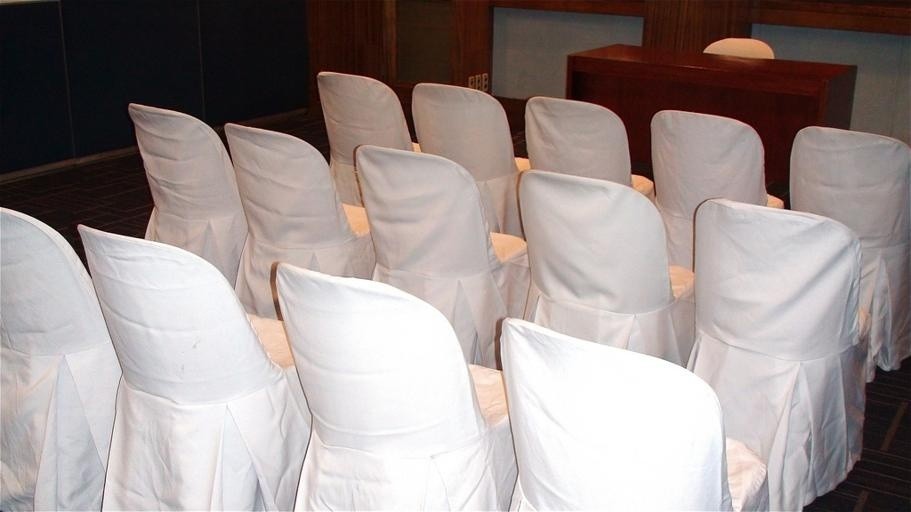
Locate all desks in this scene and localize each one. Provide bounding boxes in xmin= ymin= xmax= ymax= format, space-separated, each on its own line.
xmin=565 ymin=44 xmax=857 ymax=209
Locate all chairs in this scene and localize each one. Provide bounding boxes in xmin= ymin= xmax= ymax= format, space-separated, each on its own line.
xmin=703 ymin=38 xmax=775 ymax=60
